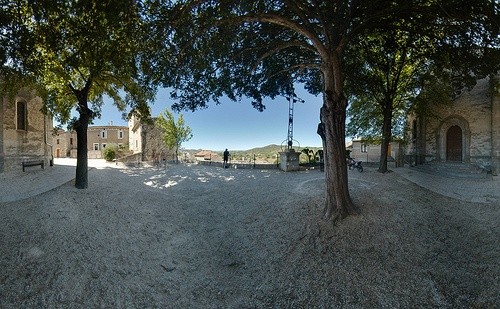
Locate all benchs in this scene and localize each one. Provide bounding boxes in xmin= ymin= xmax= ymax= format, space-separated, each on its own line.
xmin=22 ymin=160 xmax=44 ymax=172
xmin=300 ymin=161 xmax=320 ymax=169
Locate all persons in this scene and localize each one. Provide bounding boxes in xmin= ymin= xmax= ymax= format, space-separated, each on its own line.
xmin=223 ymin=148 xmax=229 ymax=168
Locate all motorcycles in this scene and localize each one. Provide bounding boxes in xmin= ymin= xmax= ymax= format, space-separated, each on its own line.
xmin=348 ymin=157 xmax=364 ymax=173
